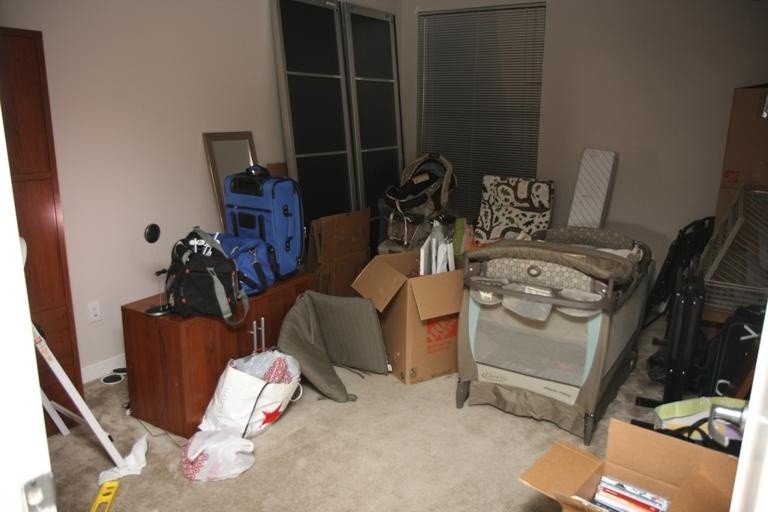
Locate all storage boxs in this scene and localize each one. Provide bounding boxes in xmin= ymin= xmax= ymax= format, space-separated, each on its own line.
xmin=517 ymin=416 xmax=739 ymax=511
xmin=350 ymin=248 xmax=464 ymax=387
xmin=699 ymin=83 xmax=768 ymax=323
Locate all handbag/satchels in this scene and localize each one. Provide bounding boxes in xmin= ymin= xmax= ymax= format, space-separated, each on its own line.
xmin=383 ymin=152 xmax=458 ymax=224
xmin=196 ymin=349 xmax=303 ymax=438
xmin=211 ymin=231 xmax=277 ymax=295
xmin=164 ymin=236 xmax=238 ymax=319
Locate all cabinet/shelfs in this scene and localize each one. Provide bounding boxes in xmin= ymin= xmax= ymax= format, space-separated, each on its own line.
xmin=120 ymin=274 xmax=317 ymax=440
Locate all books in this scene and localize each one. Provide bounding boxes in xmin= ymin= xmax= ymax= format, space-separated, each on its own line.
xmin=593 ymin=474 xmax=671 ymax=512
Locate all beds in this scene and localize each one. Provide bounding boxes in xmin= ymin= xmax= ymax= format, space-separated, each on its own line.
xmin=456 ymin=226 xmax=652 ymax=448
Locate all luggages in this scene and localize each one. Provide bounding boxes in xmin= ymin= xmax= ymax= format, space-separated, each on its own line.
xmin=666 ymin=272 xmax=704 ymax=377
xmin=704 ymin=304 xmax=765 ymax=396
xmin=224 ymin=164 xmax=311 ymax=281
